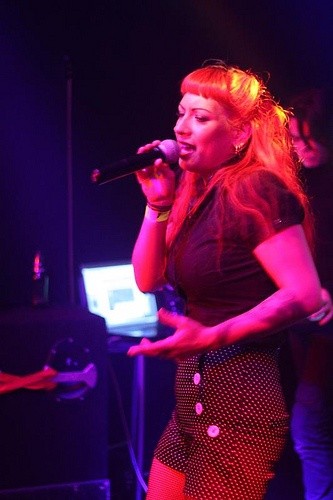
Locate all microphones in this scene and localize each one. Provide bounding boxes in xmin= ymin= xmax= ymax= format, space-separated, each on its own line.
xmin=90 ymin=139 xmax=180 ymax=186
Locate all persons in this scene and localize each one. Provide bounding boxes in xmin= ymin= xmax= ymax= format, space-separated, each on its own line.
xmin=288 ymin=77 xmax=333 ymax=500
xmin=127 ymin=65 xmax=322 ymax=500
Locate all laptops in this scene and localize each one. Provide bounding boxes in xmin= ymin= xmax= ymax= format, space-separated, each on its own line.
xmin=79 ymin=260 xmax=176 ymax=340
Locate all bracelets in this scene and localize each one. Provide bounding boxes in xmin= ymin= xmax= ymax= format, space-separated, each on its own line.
xmin=144 ymin=206 xmax=171 ymax=223
